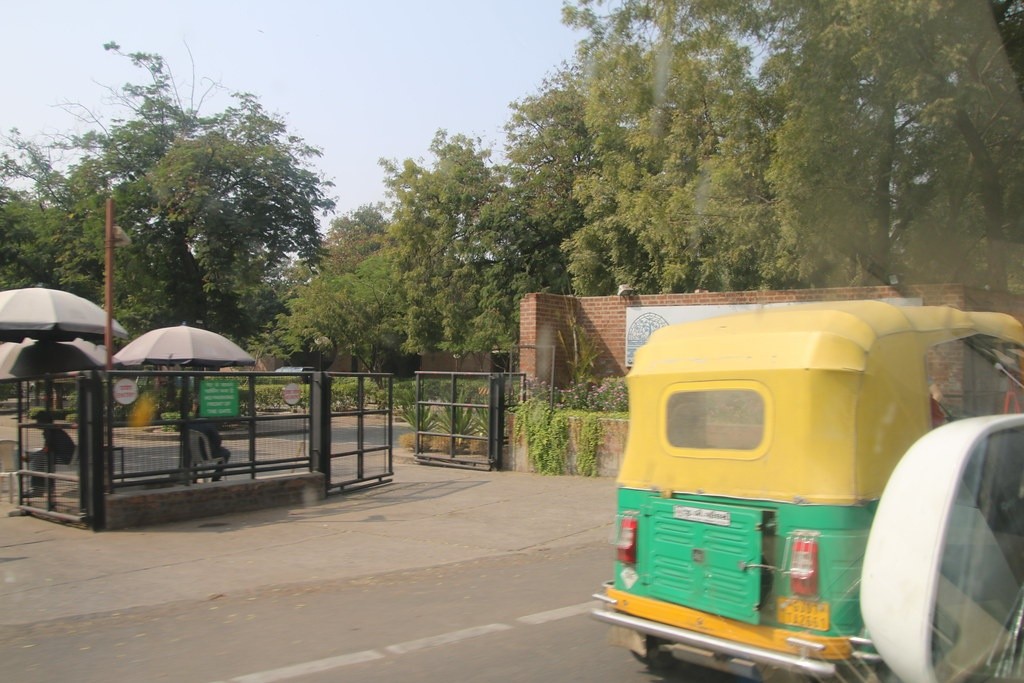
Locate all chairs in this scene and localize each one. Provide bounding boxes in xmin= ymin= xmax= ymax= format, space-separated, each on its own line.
xmin=0 ymin=440 xmax=21 ymax=505
xmin=186 ymin=429 xmax=226 ymax=485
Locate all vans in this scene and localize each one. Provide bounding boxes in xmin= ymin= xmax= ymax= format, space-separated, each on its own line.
xmin=273 ymin=367 xmax=317 ymax=379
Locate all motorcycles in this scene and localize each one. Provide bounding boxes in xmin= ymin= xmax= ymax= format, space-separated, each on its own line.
xmin=586 ymin=299 xmax=1024 ymax=683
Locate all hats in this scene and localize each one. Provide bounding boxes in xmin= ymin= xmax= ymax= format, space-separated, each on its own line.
xmin=32 ymin=411 xmax=52 ymax=421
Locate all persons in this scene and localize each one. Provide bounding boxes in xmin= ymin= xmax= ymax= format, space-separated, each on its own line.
xmin=188 ymin=420 xmax=230 ymax=482
xmin=21 ymin=412 xmax=74 ymax=499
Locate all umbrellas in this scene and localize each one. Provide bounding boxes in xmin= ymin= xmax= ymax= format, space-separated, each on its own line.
xmin=111 ymin=320 xmax=256 ymax=470
xmin=0 ymin=282 xmax=128 ymax=511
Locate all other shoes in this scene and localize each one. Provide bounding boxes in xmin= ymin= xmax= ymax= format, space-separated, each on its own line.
xmin=24 ymin=489 xmax=43 ymax=497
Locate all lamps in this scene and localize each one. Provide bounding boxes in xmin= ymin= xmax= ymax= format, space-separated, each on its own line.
xmin=617 ymin=284 xmax=634 ymax=298
